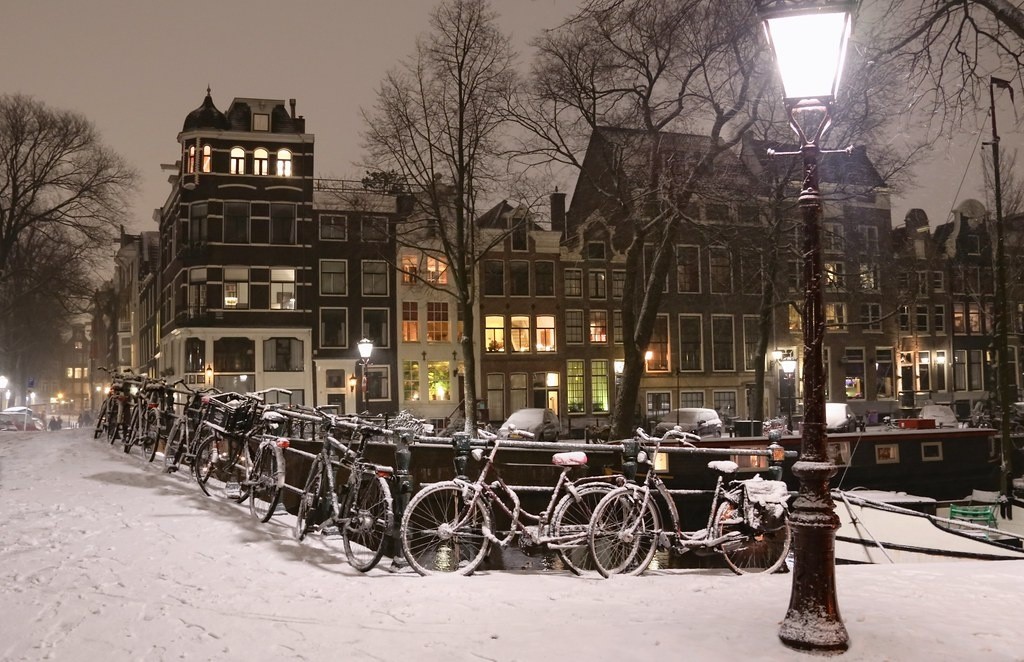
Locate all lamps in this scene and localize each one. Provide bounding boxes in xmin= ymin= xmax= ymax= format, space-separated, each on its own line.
xmin=349 ymin=374 xmax=357 ymax=392
xmin=207 ymin=364 xmax=212 ymax=378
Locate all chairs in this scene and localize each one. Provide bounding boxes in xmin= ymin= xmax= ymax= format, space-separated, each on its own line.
xmin=958 ymin=488 xmax=1001 ymax=529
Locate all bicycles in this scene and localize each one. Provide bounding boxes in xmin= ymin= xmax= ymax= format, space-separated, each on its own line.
xmin=88 ymin=366 xmax=799 ymax=578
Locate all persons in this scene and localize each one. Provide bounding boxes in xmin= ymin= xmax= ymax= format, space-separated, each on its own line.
xmin=49 ymin=418 xmax=62 ymax=431
xmin=78 ymin=410 xmax=90 ymax=428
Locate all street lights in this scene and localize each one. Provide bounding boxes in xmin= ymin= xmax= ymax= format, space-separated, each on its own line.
xmin=0 ymin=374 xmax=11 ymax=412
xmin=778 ymin=355 xmax=797 ymax=437
xmin=755 ymin=0 xmax=863 ymax=656
xmin=357 ymin=338 xmax=374 ymax=420
xmin=207 ymin=364 xmax=212 ymax=385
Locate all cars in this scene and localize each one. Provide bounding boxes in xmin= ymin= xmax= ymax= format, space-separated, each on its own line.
xmin=826 ymin=403 xmax=857 ymax=432
xmin=919 ymin=405 xmax=959 ymax=427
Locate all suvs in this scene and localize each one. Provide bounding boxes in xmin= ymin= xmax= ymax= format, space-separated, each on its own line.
xmin=499 ymin=408 xmax=560 ymax=442
xmin=655 ymin=407 xmax=723 ymax=440
